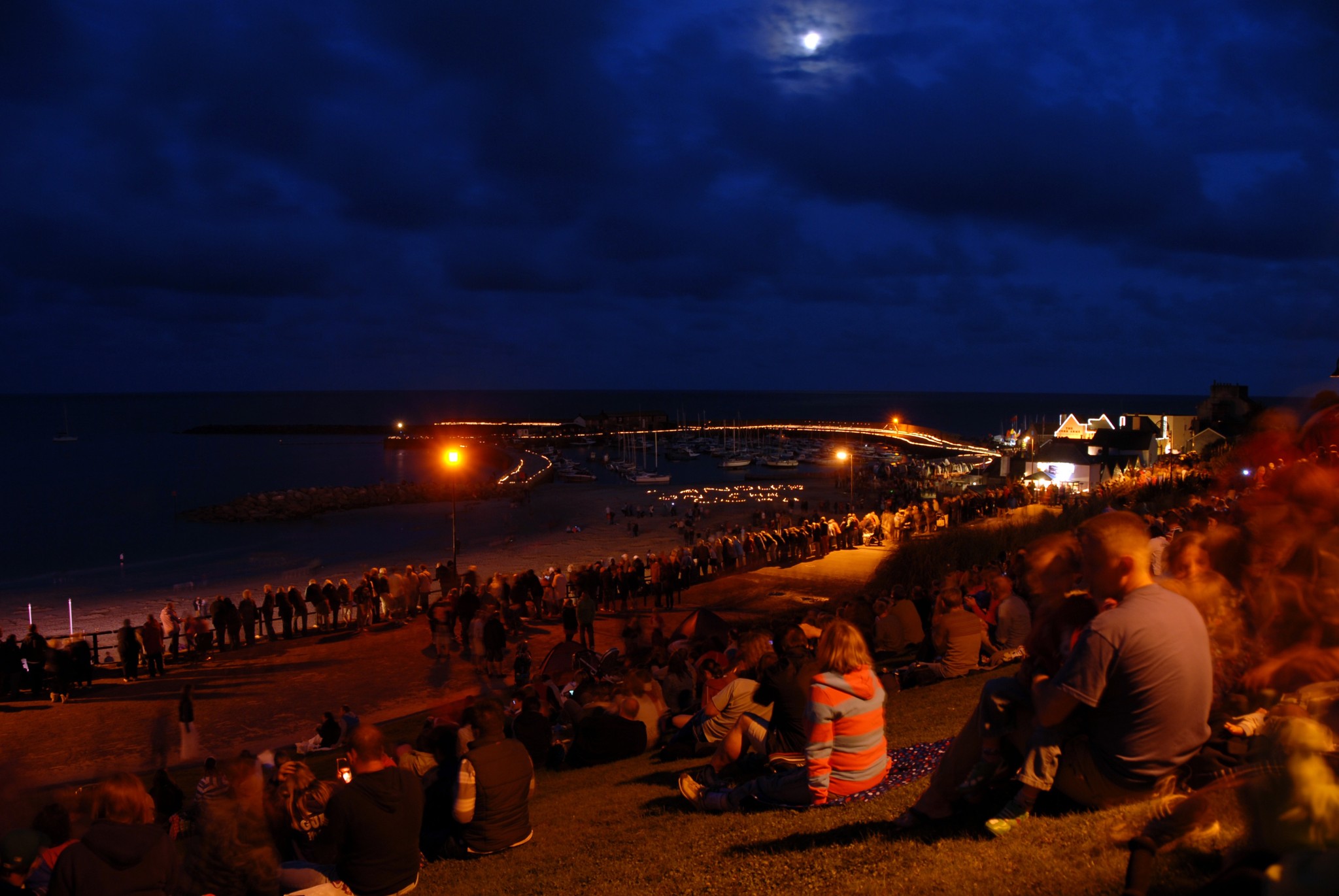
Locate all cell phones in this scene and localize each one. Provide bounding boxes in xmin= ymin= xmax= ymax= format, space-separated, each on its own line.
xmin=336 ymin=758 xmax=352 ymax=783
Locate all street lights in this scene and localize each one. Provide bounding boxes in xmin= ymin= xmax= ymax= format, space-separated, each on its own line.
xmin=837 ymin=451 xmax=853 ymax=510
xmin=1023 ymin=435 xmax=1034 ymax=503
xmin=445 ymin=447 xmax=466 ymax=561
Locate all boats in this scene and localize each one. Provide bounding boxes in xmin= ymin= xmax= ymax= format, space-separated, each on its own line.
xmin=629 ymin=470 xmax=671 ymax=482
xmin=553 ymin=459 xmax=579 ymax=470
xmin=718 ymin=458 xmax=750 ymax=466
xmin=606 ymin=462 xmax=625 ymax=469
xmin=570 ymin=435 xmax=668 ymax=458
xmin=673 ymin=435 xmax=748 ymax=459
xmin=797 ymin=454 xmax=816 ymax=462
xmin=817 ymin=458 xmax=838 ymax=464
xmin=540 ymin=446 xmax=566 ymax=462
xmin=625 ymin=469 xmax=657 ymax=480
xmin=767 ymin=460 xmax=798 ymax=466
xmin=558 ymin=471 xmax=595 ymax=482
xmin=844 ymin=441 xmax=901 ymax=455
xmin=748 ymin=433 xmax=835 ymax=464
xmin=616 ymin=464 xmax=636 ymax=472
xmin=681 ymin=449 xmax=699 ymax=460
xmin=560 ymin=466 xmax=589 ymax=474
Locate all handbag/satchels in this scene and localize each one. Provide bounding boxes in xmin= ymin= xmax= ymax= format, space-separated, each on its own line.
xmin=767 ymin=749 xmax=810 ymax=773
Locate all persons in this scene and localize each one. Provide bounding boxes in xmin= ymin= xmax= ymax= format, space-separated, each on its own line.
xmin=1 ymin=452 xmax=1338 ymax=896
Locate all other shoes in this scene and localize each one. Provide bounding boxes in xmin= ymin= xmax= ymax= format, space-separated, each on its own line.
xmin=609 ymin=609 xmax=614 ymax=612
xmin=601 ymin=608 xmax=606 ymax=611
xmin=678 ymin=773 xmax=712 ymax=813
xmin=886 ymin=807 xmax=953 ymax=836
xmin=985 ymin=800 xmax=1031 ymax=838
xmin=488 ymin=673 xmax=495 ymax=678
xmin=497 ymin=673 xmax=507 ymax=678
